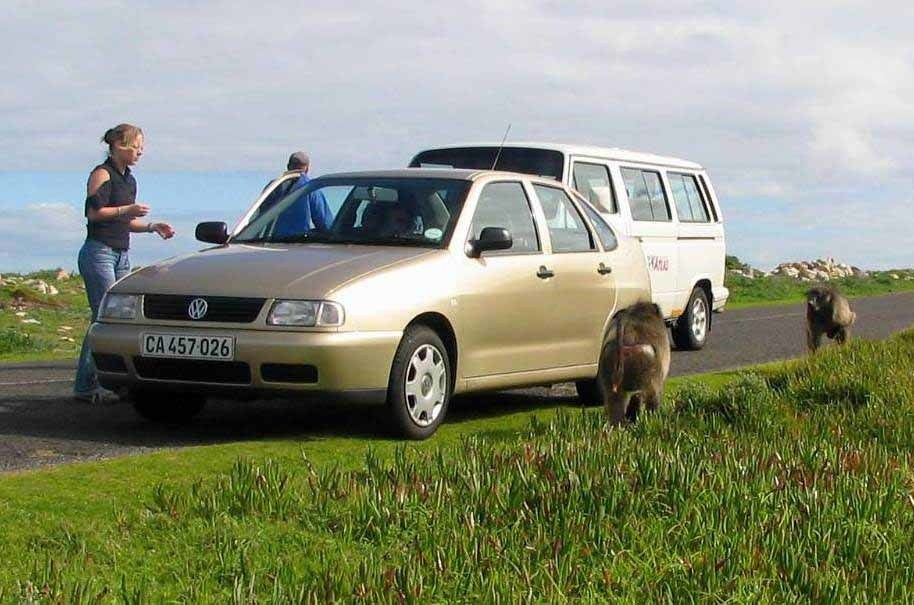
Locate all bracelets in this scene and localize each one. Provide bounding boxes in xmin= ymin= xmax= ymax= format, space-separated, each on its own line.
xmin=148 ymin=222 xmax=153 ymax=232
xmin=118 ymin=207 xmax=120 ymax=216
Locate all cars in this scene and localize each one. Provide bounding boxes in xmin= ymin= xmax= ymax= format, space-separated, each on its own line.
xmin=89 ymin=168 xmax=652 ymax=442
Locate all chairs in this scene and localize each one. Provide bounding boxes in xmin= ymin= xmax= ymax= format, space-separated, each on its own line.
xmin=353 ymin=193 xmax=407 ymax=241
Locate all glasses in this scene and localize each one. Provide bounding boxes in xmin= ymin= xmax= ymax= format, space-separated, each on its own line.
xmin=121 ymin=143 xmax=144 ymax=151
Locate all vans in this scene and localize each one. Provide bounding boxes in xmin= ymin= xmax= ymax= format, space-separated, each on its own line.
xmin=406 ymin=141 xmax=729 ymax=352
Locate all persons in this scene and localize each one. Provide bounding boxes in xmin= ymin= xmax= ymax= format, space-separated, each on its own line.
xmin=75 ymin=124 xmax=174 ymax=401
xmin=259 ymin=151 xmax=333 ymax=238
xmin=366 ymin=190 xmax=417 ymax=241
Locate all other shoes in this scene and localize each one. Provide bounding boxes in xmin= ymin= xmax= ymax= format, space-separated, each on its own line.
xmin=78 ymin=388 xmax=120 ymax=405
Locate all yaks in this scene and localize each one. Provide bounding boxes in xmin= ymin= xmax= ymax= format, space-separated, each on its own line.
xmin=806 ymin=288 xmax=857 ymax=353
xmin=598 ymin=303 xmax=669 ymax=426
